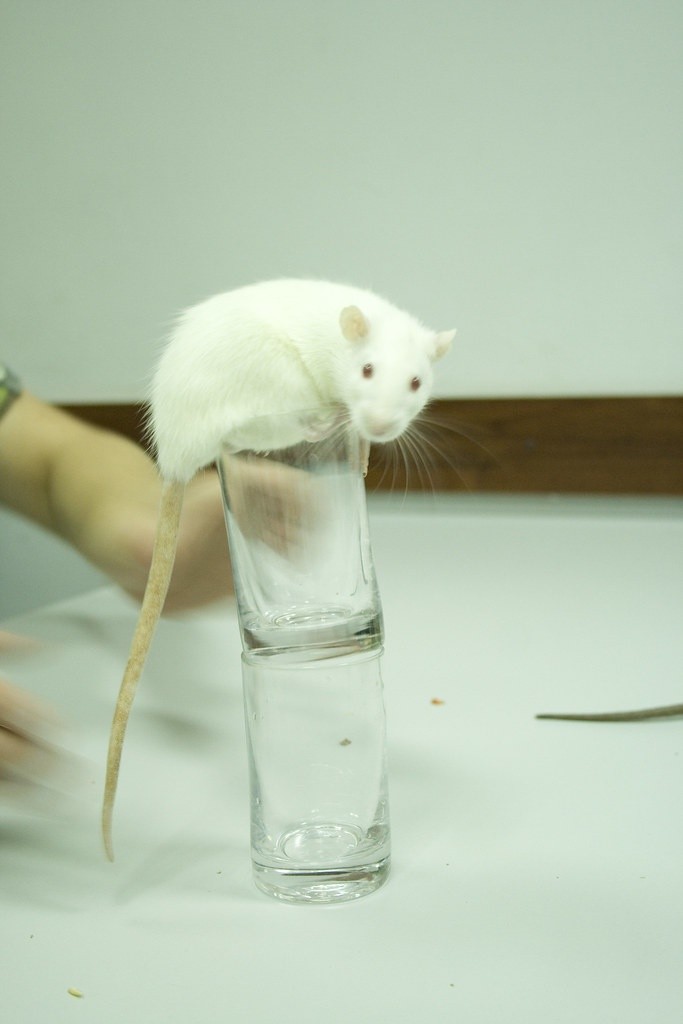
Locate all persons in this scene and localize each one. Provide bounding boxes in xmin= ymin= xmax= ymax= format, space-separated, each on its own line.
xmin=0 ymin=371 xmax=318 ymax=793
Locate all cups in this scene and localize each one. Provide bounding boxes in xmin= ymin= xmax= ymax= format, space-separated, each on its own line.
xmin=238 ymin=645 xmax=392 ymax=905
xmin=215 ymin=403 xmax=384 ymax=661
